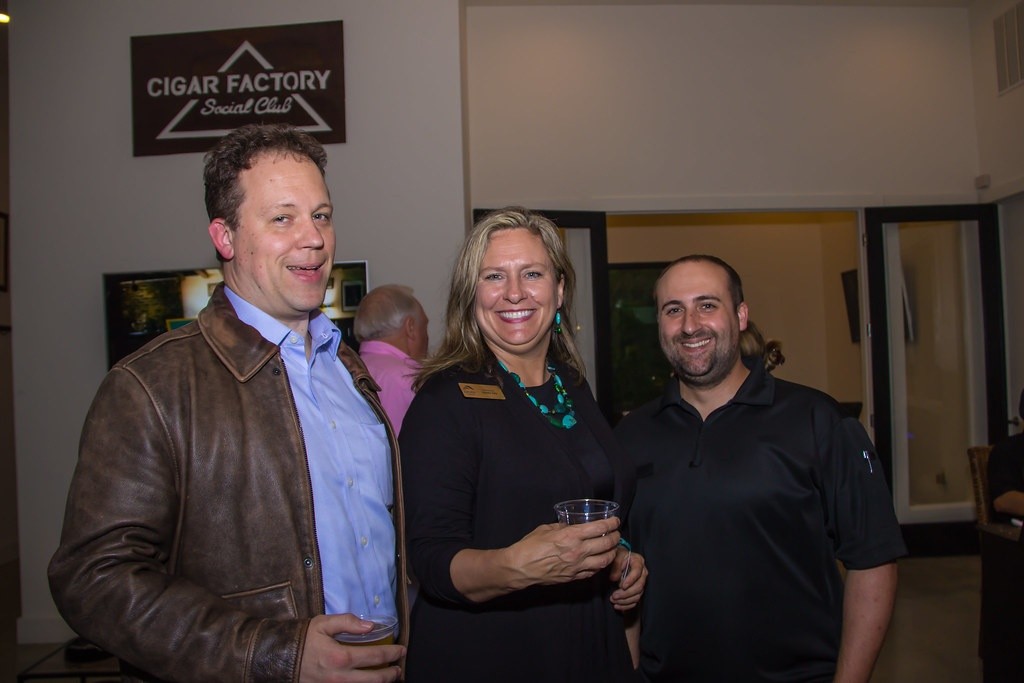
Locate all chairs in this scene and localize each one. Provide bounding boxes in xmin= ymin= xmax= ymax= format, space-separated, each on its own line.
xmin=966 ymin=445 xmax=993 ymax=541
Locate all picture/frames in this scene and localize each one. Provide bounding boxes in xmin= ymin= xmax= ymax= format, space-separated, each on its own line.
xmin=340 ymin=279 xmax=364 ymax=313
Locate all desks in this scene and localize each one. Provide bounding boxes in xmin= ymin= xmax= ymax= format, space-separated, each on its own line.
xmin=975 ymin=523 xmax=1024 ymax=661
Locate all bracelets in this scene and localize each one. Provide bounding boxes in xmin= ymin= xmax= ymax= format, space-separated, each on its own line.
xmin=619 ymin=538 xmax=631 ymax=550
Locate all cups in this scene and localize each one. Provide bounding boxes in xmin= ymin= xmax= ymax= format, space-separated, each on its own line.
xmin=332 ymin=613 xmax=398 ymax=670
xmin=553 ymin=498 xmax=620 ymax=528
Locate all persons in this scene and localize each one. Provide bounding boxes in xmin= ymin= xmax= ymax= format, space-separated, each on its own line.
xmin=48 ymin=125 xmax=408 ymax=683
xmin=354 ymin=283 xmax=429 ymax=440
xmin=397 ymin=206 xmax=649 ymax=683
xmin=985 ymin=384 xmax=1024 ymax=517
xmin=616 ymin=253 xmax=908 ymax=683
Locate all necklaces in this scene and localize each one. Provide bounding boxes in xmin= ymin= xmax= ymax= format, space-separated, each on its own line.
xmin=498 ymin=356 xmax=577 ymax=429
